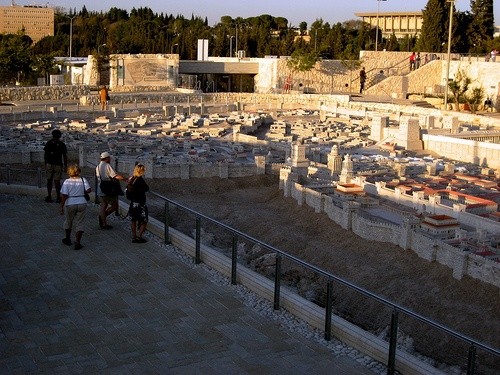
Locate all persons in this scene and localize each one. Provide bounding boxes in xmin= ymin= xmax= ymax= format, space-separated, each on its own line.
xmin=285 ymin=76 xmax=290 ymax=94
xmin=406 ymin=48 xmax=499 ymax=70
xmin=101 ymin=85 xmax=108 ymax=112
xmin=359 ymin=65 xmax=366 ymax=94
xmin=43 ymin=130 xmax=67 ymax=201
xmin=59 ymin=165 xmax=90 ymax=250
xmin=95 ymin=152 xmax=127 ymax=229
xmin=376 ymin=70 xmax=387 ymax=81
xmin=130 ymin=163 xmax=151 ymax=243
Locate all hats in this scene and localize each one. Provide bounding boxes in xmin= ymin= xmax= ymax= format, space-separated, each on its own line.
xmin=100 ymin=152 xmax=111 ymax=159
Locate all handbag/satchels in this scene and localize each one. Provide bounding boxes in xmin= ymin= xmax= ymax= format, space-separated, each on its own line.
xmin=84 ymin=191 xmax=92 ymax=202
xmin=99 ymin=180 xmax=124 ymax=199
xmin=106 ymin=94 xmax=110 ymax=101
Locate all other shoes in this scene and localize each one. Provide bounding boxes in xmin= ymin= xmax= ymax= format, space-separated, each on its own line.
xmin=45 ymin=196 xmax=53 ymax=202
xmin=100 ymin=224 xmax=113 ymax=230
xmin=74 ymin=243 xmax=82 ymax=250
xmin=135 ymin=237 xmax=148 ymax=243
xmin=56 ymin=196 xmax=62 ymax=202
xmin=98 ymin=215 xmax=103 ymax=228
xmin=62 ymin=238 xmax=72 ymax=245
xmin=132 ymin=236 xmax=136 ymax=243
